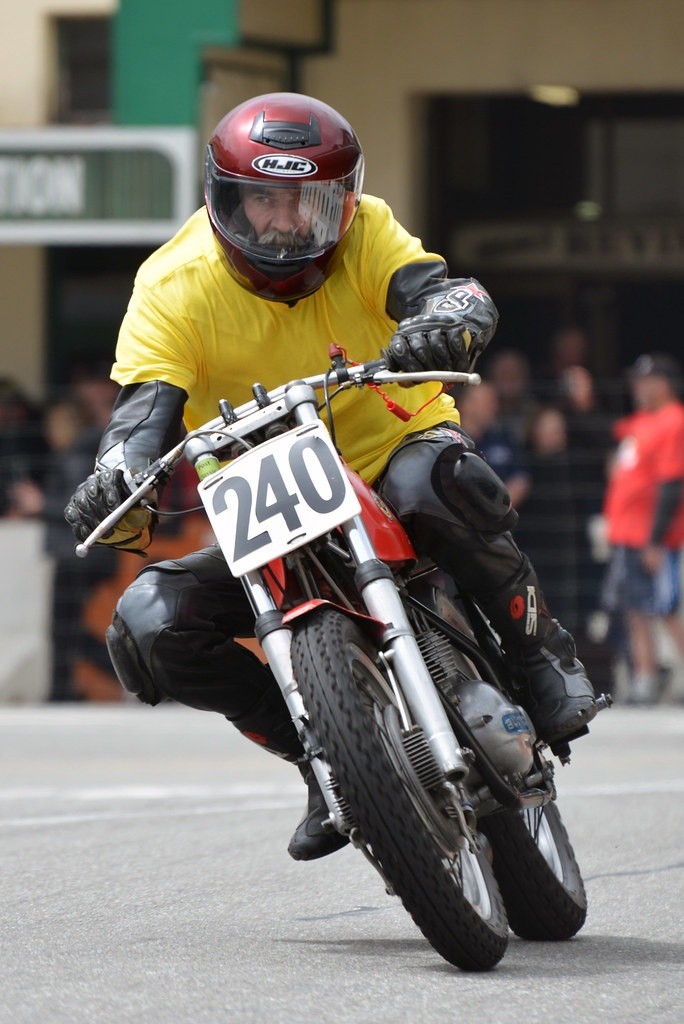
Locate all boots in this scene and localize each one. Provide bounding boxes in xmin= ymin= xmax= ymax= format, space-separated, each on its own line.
xmin=477 ymin=551 xmax=596 ymax=743
xmin=225 ymin=663 xmax=350 ymax=861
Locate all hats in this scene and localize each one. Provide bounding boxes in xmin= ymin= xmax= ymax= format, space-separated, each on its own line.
xmin=631 ymin=353 xmax=671 ymax=377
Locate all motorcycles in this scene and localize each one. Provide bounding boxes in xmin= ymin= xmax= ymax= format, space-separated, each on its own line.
xmin=68 ymin=324 xmax=613 ymax=975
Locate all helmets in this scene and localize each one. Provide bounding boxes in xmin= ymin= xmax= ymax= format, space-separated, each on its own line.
xmin=202 ymin=92 xmax=366 ymax=303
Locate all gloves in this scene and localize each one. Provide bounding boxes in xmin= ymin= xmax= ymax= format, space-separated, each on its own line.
xmin=389 ymin=313 xmax=470 ymax=383
xmin=62 ymin=467 xmax=152 ymax=553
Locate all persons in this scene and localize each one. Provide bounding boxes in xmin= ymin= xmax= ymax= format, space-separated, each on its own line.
xmin=459 ymin=323 xmax=684 ymax=703
xmin=0 ymin=371 xmax=201 ymax=701
xmin=64 ymin=93 xmax=593 ymax=863
